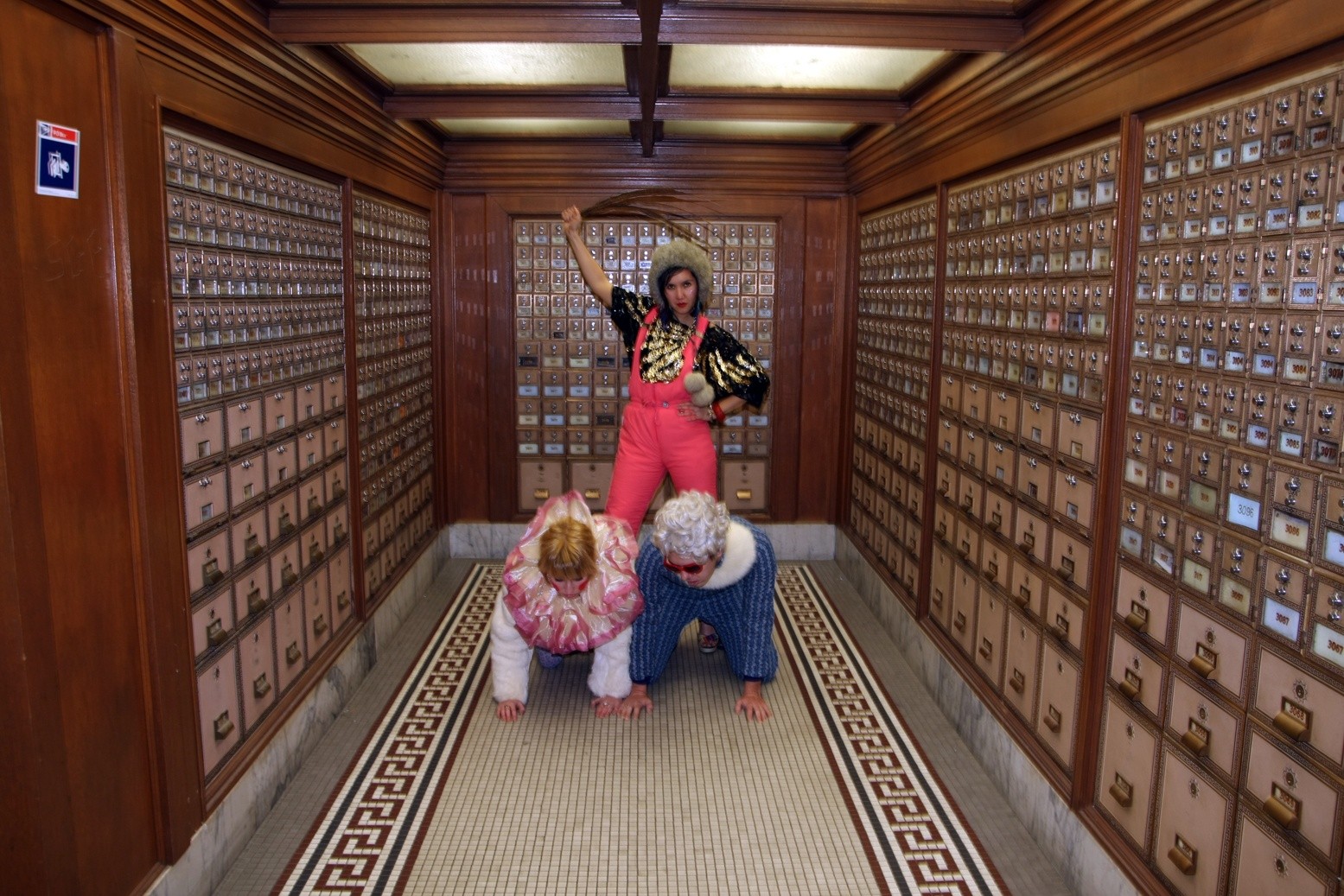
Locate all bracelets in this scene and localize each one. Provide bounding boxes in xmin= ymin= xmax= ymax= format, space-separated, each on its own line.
xmin=707 ymin=403 xmax=713 ymax=421
xmin=712 ymin=401 xmax=725 ymax=422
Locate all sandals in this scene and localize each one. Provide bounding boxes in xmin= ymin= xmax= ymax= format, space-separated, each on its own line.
xmin=696 ymin=620 xmax=719 ymax=653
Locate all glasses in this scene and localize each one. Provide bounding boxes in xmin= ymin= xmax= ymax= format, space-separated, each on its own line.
xmin=663 ymin=553 xmax=711 ymax=575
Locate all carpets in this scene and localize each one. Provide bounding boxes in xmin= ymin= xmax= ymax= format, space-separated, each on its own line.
xmin=270 ymin=564 xmax=1012 ymax=896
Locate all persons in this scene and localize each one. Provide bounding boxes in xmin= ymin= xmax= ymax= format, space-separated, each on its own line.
xmin=562 ymin=206 xmax=768 ymax=651
xmin=487 ymin=487 xmax=644 ymax=723
xmin=619 ymin=489 xmax=778 ymax=724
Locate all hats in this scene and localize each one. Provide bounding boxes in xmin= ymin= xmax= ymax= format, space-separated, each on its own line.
xmin=648 ymin=239 xmax=713 ymax=313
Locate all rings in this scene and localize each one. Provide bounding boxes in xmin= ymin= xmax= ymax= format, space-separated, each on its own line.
xmin=603 ymin=701 xmax=610 ymax=707
xmin=506 ymin=706 xmax=509 ymax=708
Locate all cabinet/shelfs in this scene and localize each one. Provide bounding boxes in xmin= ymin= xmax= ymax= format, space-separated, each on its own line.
xmin=157 ymin=39 xmax=1344 ymax=896
